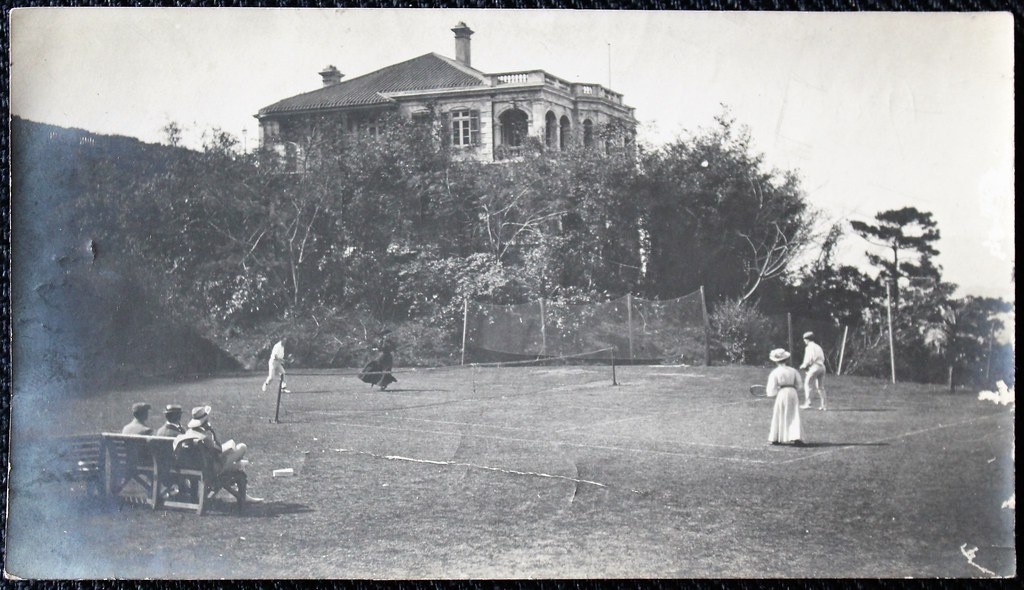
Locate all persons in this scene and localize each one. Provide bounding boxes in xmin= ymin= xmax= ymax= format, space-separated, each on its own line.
xmin=158 ymin=403 xmax=198 ymax=496
xmin=799 ymin=332 xmax=829 ymax=411
xmin=358 ymin=329 xmax=398 ymax=392
xmin=261 ymin=334 xmax=294 ymax=392
xmin=122 ymin=403 xmax=179 ymax=495
xmin=188 ymin=406 xmax=264 ymax=503
xmin=765 ymin=348 xmax=806 ymax=445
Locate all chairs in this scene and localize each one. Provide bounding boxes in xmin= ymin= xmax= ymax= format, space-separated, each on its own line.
xmin=52 ymin=435 xmax=107 ymax=508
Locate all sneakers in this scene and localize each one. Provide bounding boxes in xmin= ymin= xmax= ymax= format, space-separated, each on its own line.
xmin=281 ymin=388 xmax=291 ymax=394
xmin=262 ymin=382 xmax=269 ymax=392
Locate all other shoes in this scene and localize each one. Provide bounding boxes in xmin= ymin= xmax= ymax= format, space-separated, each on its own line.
xmin=800 ymin=405 xmax=812 ymax=409
xmin=771 ymin=441 xmax=781 ymax=445
xmin=794 ymin=441 xmax=804 ymax=445
xmin=245 ymin=494 xmax=264 ymax=505
xmin=819 ymin=407 xmax=828 ymax=411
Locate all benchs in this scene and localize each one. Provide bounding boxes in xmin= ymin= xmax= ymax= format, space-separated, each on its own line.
xmin=103 ymin=432 xmax=246 ymax=518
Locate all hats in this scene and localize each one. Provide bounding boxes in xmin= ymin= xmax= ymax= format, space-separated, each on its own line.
xmin=187 ymin=405 xmax=212 ymax=428
xmin=768 ymin=348 xmax=790 ymax=362
xmin=163 ymin=404 xmax=183 ymax=414
xmin=803 ymin=332 xmax=815 ymax=339
xmin=132 ymin=402 xmax=153 ymax=413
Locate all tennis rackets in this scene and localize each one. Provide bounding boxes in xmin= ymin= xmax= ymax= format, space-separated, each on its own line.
xmin=285 ymin=353 xmax=296 ymax=365
xmin=749 ymin=384 xmax=767 ymax=397
